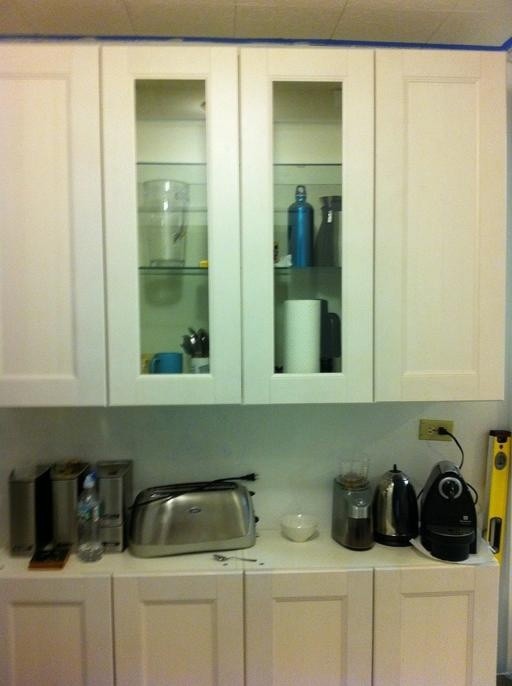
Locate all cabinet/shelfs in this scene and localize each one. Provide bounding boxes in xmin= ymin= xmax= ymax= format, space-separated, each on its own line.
xmin=0 ymin=573 xmax=115 ymax=686
xmin=2 ymin=36 xmax=110 ymax=412
xmin=375 ymin=562 xmax=501 ymax=685
xmin=244 ymin=566 xmax=375 ymax=686
xmin=375 ymin=45 xmax=510 ymax=404
xmin=101 ymin=40 xmax=376 ymax=410
xmin=114 ymin=569 xmax=244 ymax=686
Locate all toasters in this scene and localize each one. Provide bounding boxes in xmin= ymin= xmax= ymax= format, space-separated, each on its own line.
xmin=128 ymin=482 xmax=260 ymax=559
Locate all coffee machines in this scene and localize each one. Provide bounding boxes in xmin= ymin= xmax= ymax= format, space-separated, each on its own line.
xmin=420 ymin=460 xmax=479 ymax=561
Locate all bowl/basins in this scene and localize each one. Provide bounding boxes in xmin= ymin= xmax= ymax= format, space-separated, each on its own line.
xmin=279 ymin=514 xmax=320 ymax=542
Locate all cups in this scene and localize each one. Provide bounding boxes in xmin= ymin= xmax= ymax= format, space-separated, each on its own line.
xmin=150 ymin=351 xmax=184 ymax=374
xmin=142 ymin=179 xmax=192 ymax=267
xmin=189 ymin=355 xmax=210 ymax=373
xmin=282 ymin=298 xmax=341 ymax=373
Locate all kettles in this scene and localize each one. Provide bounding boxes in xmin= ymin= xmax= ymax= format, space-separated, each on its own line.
xmin=375 ymin=462 xmax=418 ymax=546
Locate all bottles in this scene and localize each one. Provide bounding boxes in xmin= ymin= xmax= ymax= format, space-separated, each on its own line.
xmin=330 ymin=195 xmax=341 ymax=268
xmin=286 ymin=183 xmax=314 ymax=266
xmin=78 ymin=474 xmax=103 ymax=562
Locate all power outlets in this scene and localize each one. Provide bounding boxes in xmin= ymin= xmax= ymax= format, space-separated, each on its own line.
xmin=416 ymin=418 xmax=455 ymax=442
